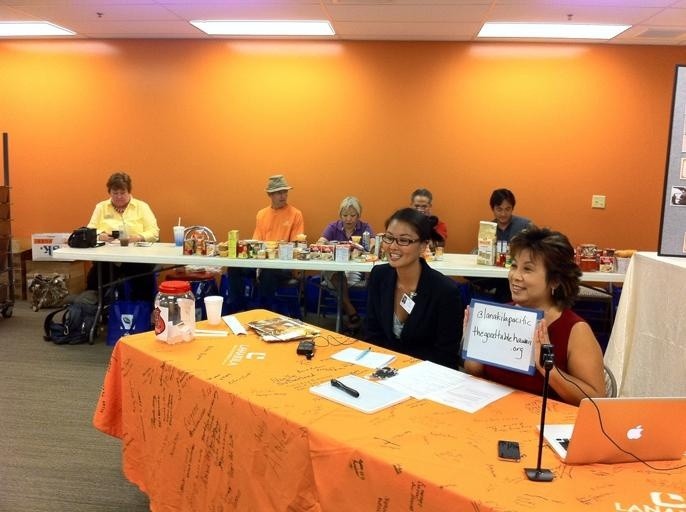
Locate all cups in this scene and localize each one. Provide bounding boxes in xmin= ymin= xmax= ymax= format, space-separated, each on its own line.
xmin=615 ymin=257 xmax=629 ymax=273
xmin=174 ymin=227 xmax=186 ymax=245
xmin=351 ymin=236 xmax=361 ymax=243
xmin=118 ymin=226 xmax=130 ymax=246
xmin=434 ymin=242 xmax=444 ymax=260
xmin=202 ymin=295 xmax=224 ymax=324
xmin=266 ymin=241 xmax=276 ymax=259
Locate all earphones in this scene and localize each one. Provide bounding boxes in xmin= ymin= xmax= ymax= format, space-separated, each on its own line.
xmin=306 ymin=352 xmax=314 ymax=360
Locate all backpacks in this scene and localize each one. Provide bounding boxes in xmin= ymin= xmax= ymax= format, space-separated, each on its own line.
xmin=44 ymin=305 xmax=97 ymax=344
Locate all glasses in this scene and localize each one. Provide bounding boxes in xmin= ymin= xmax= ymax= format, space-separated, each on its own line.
xmin=381 ymin=234 xmax=420 ymax=246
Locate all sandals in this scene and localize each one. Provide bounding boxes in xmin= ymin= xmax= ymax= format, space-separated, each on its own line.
xmin=349 ymin=314 xmax=360 ymax=323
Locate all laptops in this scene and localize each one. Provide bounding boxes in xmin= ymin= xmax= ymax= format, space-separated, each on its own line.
xmin=536 ymin=397 xmax=686 ymax=465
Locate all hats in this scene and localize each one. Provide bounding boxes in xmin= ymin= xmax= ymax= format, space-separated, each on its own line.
xmin=266 ymin=175 xmax=292 ymax=192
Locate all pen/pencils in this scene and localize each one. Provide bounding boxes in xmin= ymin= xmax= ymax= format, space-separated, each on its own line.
xmin=356 ymin=346 xmax=371 ymax=360
xmin=331 ymin=378 xmax=359 ymax=398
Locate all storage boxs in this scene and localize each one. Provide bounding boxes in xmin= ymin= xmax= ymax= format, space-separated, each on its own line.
xmin=24 ymin=267 xmax=86 ymax=309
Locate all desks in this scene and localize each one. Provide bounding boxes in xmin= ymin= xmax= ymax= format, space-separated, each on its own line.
xmin=29 ymin=234 xmax=635 ymax=337
xmin=113 ymin=308 xmax=686 ymax=510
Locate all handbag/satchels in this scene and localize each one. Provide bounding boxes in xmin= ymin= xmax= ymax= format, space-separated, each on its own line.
xmin=29 ymin=275 xmax=69 ymax=311
xmin=108 ymin=301 xmax=151 ymax=345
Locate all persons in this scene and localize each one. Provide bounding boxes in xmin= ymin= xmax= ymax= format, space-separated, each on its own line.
xmin=408 ymin=188 xmax=449 ymax=251
xmin=224 ymin=175 xmax=304 ymax=315
xmin=469 ymin=188 xmax=537 ymax=302
xmin=316 ymin=196 xmax=375 ymax=325
xmin=361 ymin=208 xmax=464 ymax=376
xmin=465 ymin=224 xmax=607 ymax=407
xmin=84 ymin=171 xmax=159 ymax=303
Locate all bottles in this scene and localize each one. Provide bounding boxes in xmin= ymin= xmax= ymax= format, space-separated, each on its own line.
xmin=494 ymin=242 xmax=512 ymax=268
xmin=153 ymin=280 xmax=195 ymax=345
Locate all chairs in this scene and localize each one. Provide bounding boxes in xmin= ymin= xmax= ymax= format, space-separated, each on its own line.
xmin=602 ymin=365 xmax=619 ymax=397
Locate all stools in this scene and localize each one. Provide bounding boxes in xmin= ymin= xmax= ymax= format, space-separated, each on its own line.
xmin=187 ymin=277 xmax=468 ymax=321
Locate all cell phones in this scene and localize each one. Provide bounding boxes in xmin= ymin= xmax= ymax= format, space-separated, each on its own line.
xmin=296 ymin=340 xmax=315 ymax=360
xmin=498 ymin=440 xmax=520 ymax=462
xmin=112 ymin=230 xmax=119 ymax=240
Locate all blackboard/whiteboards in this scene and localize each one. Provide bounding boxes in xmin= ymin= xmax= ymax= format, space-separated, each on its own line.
xmin=462 ymin=298 xmax=544 ymax=376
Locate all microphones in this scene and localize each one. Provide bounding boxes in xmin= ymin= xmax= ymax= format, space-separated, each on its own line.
xmin=523 ymin=344 xmax=555 ymax=482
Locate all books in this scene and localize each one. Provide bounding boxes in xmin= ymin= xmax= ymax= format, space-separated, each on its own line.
xmin=249 ymin=316 xmax=318 ymax=343
